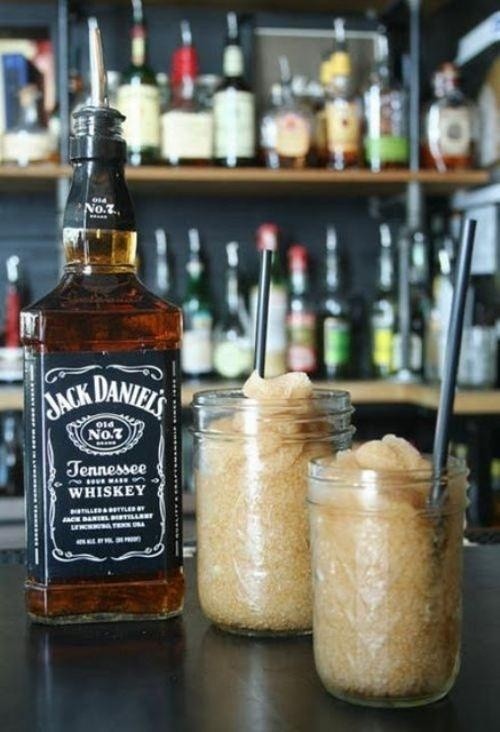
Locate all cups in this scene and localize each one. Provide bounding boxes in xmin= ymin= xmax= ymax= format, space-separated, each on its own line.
xmin=190 ymin=386 xmax=357 ymax=641
xmin=306 ymin=450 xmax=471 ymax=710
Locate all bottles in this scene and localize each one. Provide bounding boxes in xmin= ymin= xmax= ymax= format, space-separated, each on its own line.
xmin=0 ymin=222 xmax=496 ymax=385
xmin=16 ymin=105 xmax=188 ymax=627
xmin=2 ymin=0 xmax=484 ymax=173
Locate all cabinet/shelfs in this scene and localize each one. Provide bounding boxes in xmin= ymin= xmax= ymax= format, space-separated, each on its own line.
xmin=0 ymin=164 xmax=500 ymax=414
